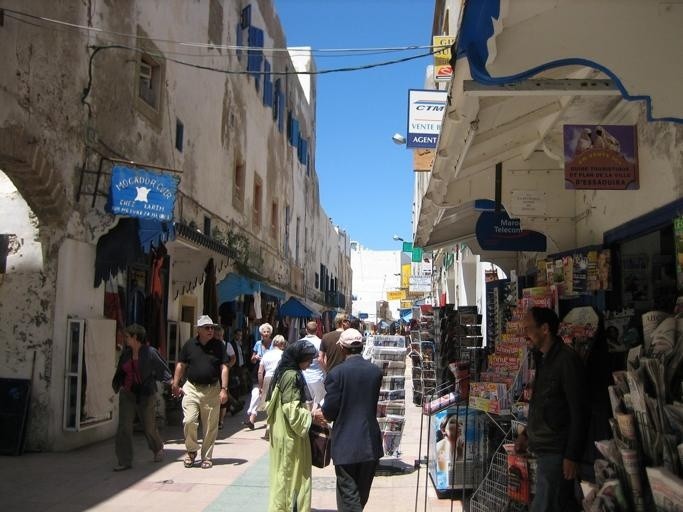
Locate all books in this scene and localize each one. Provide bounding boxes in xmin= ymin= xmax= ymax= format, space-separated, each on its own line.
xmin=533 ymin=247 xmax=599 ymax=301
xmin=578 ymin=294 xmax=683 ymax=511
xmin=361 ymin=282 xmax=560 ymax=459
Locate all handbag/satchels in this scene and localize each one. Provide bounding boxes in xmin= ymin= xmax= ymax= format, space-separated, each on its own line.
xmin=309 ymin=422 xmax=331 ymax=468
xmin=131 ymin=383 xmax=153 ymax=396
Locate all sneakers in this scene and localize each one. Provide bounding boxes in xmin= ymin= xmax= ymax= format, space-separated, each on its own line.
xmin=114 ymin=464 xmax=132 ymax=471
xmin=244 ymin=419 xmax=254 ymax=429
xmin=184 ymin=452 xmax=197 ymax=468
xmin=219 ymin=423 xmax=224 ymax=429
xmin=201 ymin=461 xmax=212 ymax=468
xmin=154 ymin=450 xmax=165 ymax=462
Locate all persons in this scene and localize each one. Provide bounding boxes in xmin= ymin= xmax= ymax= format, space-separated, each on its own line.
xmin=110 ymin=323 xmax=183 ymax=471
xmin=594 ymin=250 xmax=609 ymax=290
xmin=170 ymin=314 xmax=231 ymax=469
xmin=263 ymin=339 xmax=332 ymax=512
xmin=320 ymin=327 xmax=385 ymax=512
xmin=575 ymin=125 xmax=622 ymax=158
xmin=434 ymin=411 xmax=467 ymax=475
xmin=511 ymin=304 xmax=593 ymax=512
xmin=598 ymin=262 xmax=613 ymax=290
xmin=212 ymin=311 xmax=380 ymax=443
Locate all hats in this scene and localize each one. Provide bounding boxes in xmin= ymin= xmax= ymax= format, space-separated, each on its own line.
xmin=123 ymin=324 xmax=145 ymax=337
xmin=197 ymin=315 xmax=218 ymax=326
xmin=336 ymin=327 xmax=363 ymax=348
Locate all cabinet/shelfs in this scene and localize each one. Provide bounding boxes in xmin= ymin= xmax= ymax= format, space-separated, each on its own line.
xmin=361 ymin=335 xmax=407 ymax=473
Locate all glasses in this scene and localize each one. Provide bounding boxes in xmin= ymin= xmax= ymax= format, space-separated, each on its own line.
xmin=203 ymin=326 xmax=214 ymax=330
